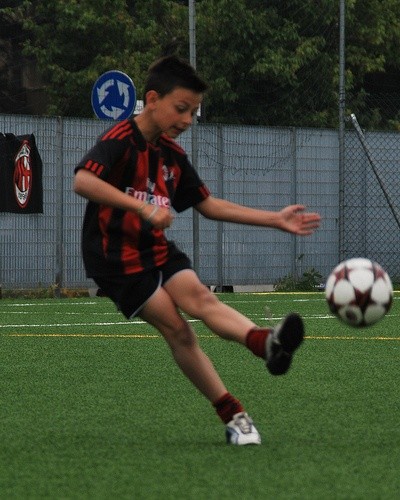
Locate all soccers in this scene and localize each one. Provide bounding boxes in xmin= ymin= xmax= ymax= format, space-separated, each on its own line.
xmin=325 ymin=257 xmax=394 ymax=328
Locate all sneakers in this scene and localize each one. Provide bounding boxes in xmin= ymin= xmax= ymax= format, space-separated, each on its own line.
xmin=266 ymin=313 xmax=305 ymax=376
xmin=225 ymin=412 xmax=261 ymax=445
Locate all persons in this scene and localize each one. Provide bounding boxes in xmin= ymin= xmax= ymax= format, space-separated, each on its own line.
xmin=71 ymin=55 xmax=322 ymax=446
